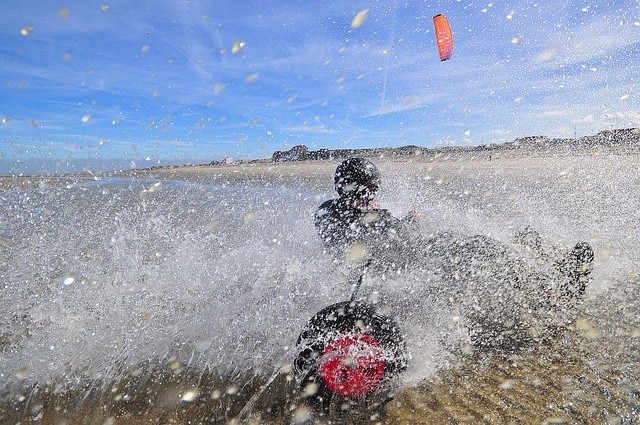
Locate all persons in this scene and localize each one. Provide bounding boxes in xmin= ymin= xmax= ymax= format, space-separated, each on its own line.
xmin=314 ymin=157 xmax=596 ymax=343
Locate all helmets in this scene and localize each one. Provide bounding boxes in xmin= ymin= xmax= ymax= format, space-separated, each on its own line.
xmin=335 ymin=157 xmax=378 ymax=193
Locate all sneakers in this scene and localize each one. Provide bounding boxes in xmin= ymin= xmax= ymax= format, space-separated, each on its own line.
xmin=560 ymin=242 xmax=594 ymax=299
